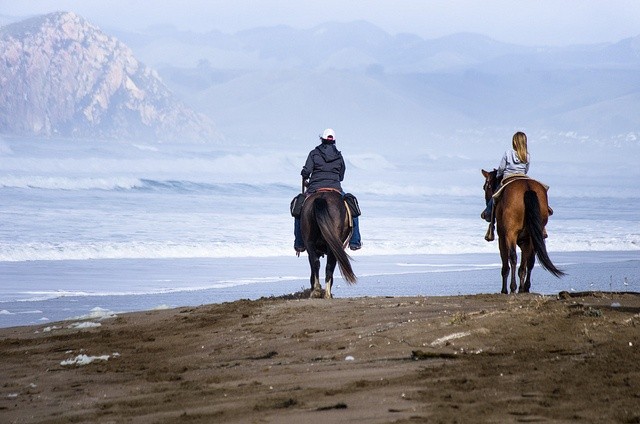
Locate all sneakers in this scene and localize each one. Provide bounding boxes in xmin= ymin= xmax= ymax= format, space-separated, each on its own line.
xmin=481 ymin=210 xmax=491 ymax=222
xmin=350 ymin=242 xmax=361 ymax=250
xmin=296 ymin=247 xmax=305 ymax=251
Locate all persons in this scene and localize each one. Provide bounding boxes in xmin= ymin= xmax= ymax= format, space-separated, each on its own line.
xmin=485 ymin=131 xmax=530 ymax=221
xmin=294 ymin=127 xmax=361 ymax=250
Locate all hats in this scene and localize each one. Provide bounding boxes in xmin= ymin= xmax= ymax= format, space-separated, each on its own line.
xmin=319 ymin=129 xmax=336 ymax=141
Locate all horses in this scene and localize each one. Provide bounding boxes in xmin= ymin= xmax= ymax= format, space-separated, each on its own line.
xmin=481 ymin=167 xmax=572 ymax=295
xmin=301 ymin=191 xmax=359 ymax=300
